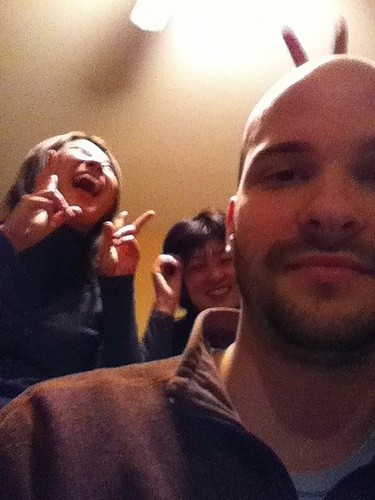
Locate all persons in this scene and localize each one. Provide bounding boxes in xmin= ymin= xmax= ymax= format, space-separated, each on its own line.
xmin=0 ymin=17 xmax=350 ymax=405
xmin=0 ymin=56 xmax=374 ymax=500
